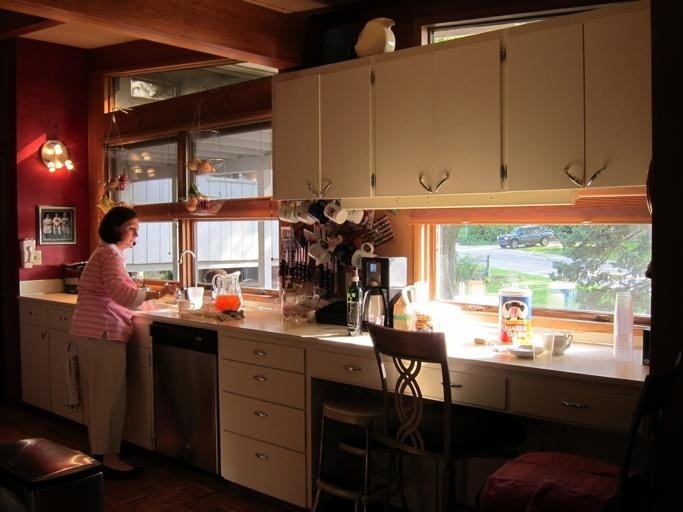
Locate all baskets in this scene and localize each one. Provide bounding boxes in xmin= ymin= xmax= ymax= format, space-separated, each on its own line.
xmin=179 ymin=195 xmax=227 ymax=216
xmin=101 ymin=148 xmax=127 ymax=160
xmin=98 ymin=204 xmax=135 ymax=214
xmin=184 ymin=159 xmax=224 ymax=174
xmin=98 ymin=175 xmax=129 ymax=191
xmin=62 ymin=262 xmax=86 ymax=293
xmin=189 ymin=129 xmax=218 ymax=144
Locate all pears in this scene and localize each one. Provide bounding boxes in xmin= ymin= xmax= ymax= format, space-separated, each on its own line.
xmin=188 ymin=157 xmax=201 ymax=171
xmin=196 ymin=160 xmax=211 ymax=172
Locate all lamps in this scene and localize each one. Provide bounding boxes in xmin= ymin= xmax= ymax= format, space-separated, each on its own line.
xmin=41 ymin=138 xmax=75 ymax=172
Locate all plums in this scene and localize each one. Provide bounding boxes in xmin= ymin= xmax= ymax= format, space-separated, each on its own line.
xmin=119 ymin=174 xmax=128 ymax=182
xmin=117 ymin=183 xmax=125 ymax=190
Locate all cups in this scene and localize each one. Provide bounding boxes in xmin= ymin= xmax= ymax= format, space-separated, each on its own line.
xmin=544 ymin=333 xmax=573 ymax=355
xmin=350 ymin=243 xmax=378 ymax=269
xmin=295 ymin=200 xmax=315 ymax=225
xmin=178 ymin=300 xmax=195 ymax=312
xmin=188 ymin=287 xmax=204 ymax=310
xmin=307 ymin=199 xmax=329 ymax=224
xmin=324 ymin=200 xmax=348 ymax=225
xmin=531 ymin=330 xmax=554 ymax=363
xmin=347 ymin=210 xmax=364 ymax=224
xmin=278 ymin=200 xmax=299 ymax=224
xmin=613 ymin=292 xmax=633 ymax=363
xmin=307 ymin=239 xmax=331 ymax=265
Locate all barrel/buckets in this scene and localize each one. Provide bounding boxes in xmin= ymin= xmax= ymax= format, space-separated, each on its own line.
xmin=178 ymin=250 xmax=198 ymax=287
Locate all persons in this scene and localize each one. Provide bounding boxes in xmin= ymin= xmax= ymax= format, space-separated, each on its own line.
xmin=505 ymin=302 xmax=524 ymax=320
xmin=43 ymin=211 xmax=69 ymax=239
xmin=69 ymin=206 xmax=174 ymax=481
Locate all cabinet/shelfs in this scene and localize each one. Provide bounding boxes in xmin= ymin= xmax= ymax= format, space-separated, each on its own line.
xmin=122 ymin=316 xmax=155 ymax=452
xmin=222 ymin=328 xmax=310 ymax=510
xmin=19 ymin=281 xmax=87 ymax=425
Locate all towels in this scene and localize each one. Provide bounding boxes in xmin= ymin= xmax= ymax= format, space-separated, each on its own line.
xmin=62 ymin=338 xmax=79 ymax=408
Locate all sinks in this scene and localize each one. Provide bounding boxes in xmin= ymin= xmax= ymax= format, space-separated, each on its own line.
xmin=134 ymin=301 xmax=174 ymax=313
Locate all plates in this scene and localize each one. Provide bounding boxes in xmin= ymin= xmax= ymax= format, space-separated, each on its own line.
xmin=508 ymin=346 xmax=542 ymax=358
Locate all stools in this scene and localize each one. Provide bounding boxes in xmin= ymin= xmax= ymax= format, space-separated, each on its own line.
xmin=0 ymin=435 xmax=107 ymax=512
xmin=314 ymin=401 xmax=394 ymax=511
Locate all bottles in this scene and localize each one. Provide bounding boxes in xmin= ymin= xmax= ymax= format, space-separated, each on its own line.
xmin=281 ymin=287 xmax=297 ymax=320
xmin=347 ymin=266 xmax=364 ymax=336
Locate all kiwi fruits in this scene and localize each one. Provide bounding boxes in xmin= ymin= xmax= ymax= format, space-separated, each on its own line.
xmin=110 ymin=177 xmax=119 ymax=186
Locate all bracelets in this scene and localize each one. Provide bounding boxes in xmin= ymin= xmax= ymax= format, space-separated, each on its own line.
xmin=157 ymin=290 xmax=160 ymax=298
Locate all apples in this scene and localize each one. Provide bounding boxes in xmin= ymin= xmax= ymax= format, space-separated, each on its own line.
xmin=200 ymin=199 xmax=212 ymax=210
xmin=185 ymin=197 xmax=198 ymax=211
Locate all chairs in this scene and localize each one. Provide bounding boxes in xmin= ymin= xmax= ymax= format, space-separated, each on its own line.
xmin=360 ymin=321 xmax=494 ymax=511
xmin=472 ymin=374 xmax=653 ymax=511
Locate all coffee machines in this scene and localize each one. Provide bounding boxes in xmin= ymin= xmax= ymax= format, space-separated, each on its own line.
xmin=360 ymin=257 xmax=408 ymax=332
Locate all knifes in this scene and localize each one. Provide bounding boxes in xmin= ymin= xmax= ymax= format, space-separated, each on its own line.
xmin=294 ymin=247 xmax=306 ymax=282
xmin=285 ymin=249 xmax=288 ymax=281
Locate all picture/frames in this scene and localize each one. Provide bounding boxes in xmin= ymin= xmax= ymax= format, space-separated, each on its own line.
xmin=38 ymin=206 xmax=77 ymax=244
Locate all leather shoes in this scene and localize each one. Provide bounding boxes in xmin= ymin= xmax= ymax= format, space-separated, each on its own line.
xmin=101 ymin=465 xmax=146 ymax=479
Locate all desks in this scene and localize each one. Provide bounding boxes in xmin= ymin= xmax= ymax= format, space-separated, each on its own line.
xmin=307 ymin=337 xmax=650 ymax=511
